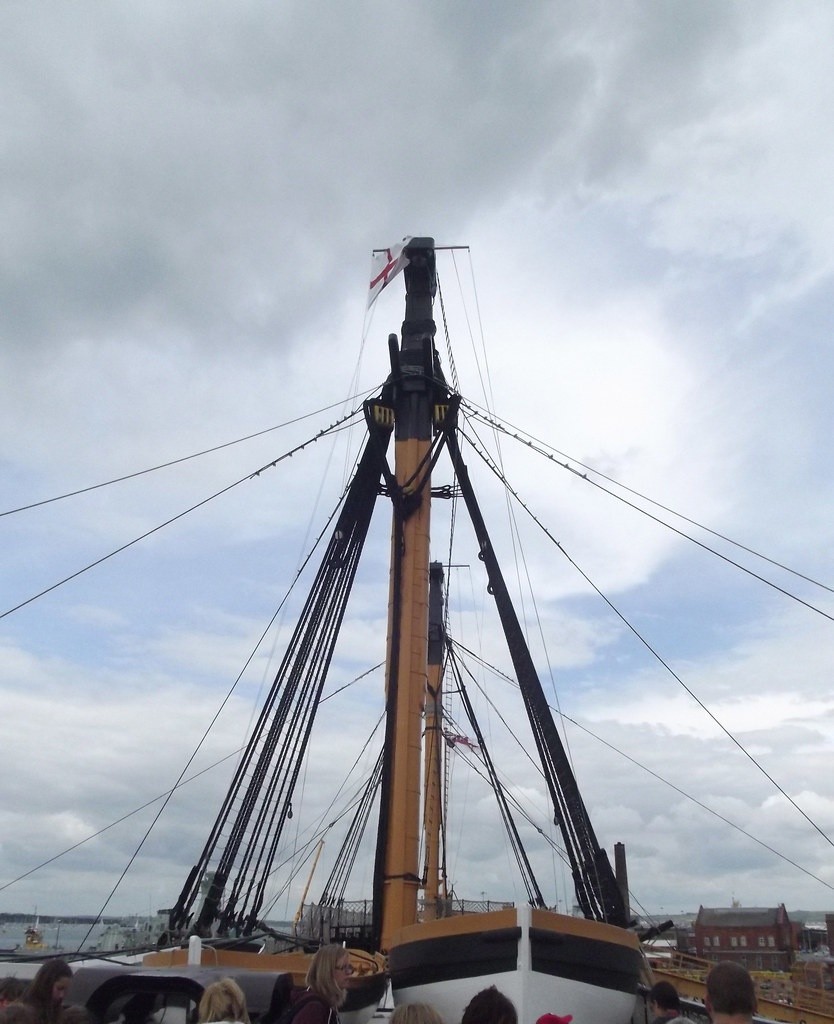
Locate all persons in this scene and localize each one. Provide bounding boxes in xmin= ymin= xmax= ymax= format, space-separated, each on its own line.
xmin=650 ymin=981 xmax=695 ymax=1024
xmin=704 ymin=960 xmax=758 ymax=1024
xmin=198 ymin=977 xmax=251 ymax=1024
xmin=536 ymin=1013 xmax=573 ymax=1024
xmin=0 ymin=958 xmax=92 ymax=1024
xmin=388 ymin=1001 xmax=443 ymax=1024
xmin=287 ymin=944 xmax=351 ymax=1024
xmin=461 ymin=985 xmax=518 ymax=1024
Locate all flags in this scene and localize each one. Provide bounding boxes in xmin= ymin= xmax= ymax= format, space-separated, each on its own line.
xmin=447 ymin=731 xmax=482 ymax=756
xmin=366 ymin=235 xmax=415 ymax=311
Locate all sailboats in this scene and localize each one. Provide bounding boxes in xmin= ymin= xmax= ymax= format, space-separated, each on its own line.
xmin=1 ymin=236 xmax=834 ymax=1024
